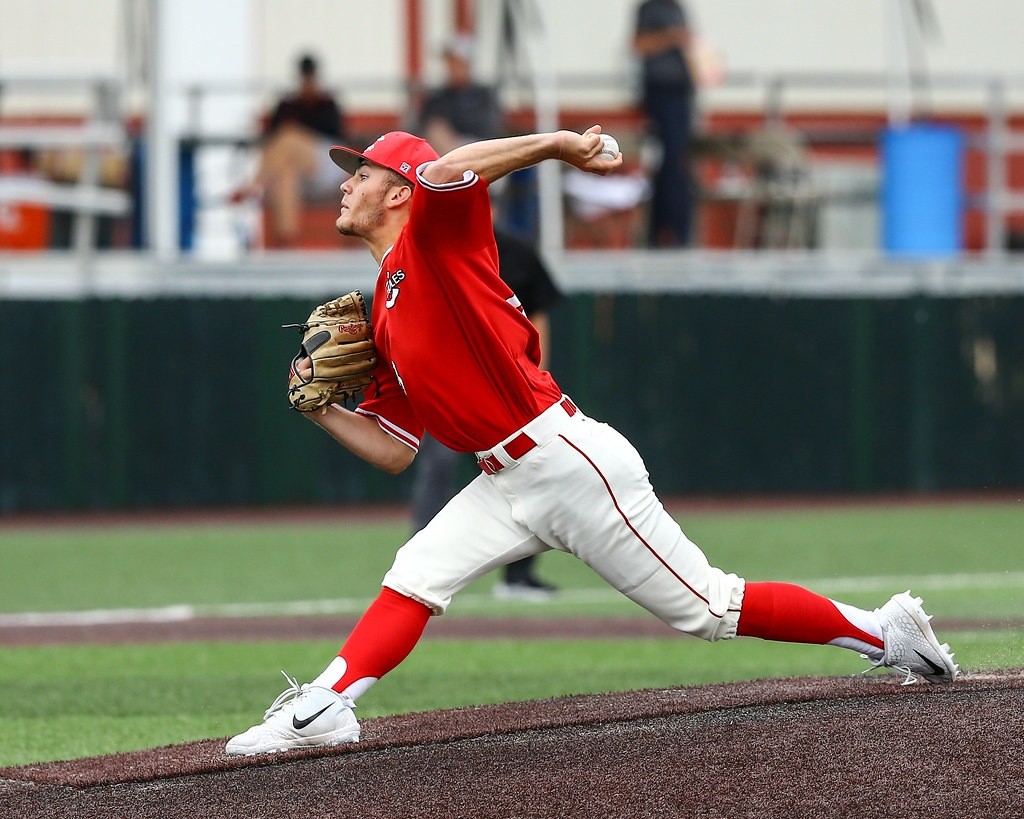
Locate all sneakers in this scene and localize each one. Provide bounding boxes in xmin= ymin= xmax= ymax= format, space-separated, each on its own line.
xmin=859 ymin=590 xmax=960 ymax=685
xmin=225 ymin=670 xmax=361 ymax=756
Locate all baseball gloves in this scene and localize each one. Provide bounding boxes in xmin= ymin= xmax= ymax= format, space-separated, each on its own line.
xmin=280 ymin=287 xmax=378 ymax=416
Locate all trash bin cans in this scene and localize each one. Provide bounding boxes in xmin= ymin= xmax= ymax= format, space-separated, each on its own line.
xmin=876 ymin=121 xmax=966 ymax=264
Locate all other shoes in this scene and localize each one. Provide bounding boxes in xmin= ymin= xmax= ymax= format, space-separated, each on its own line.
xmin=495 ymin=578 xmax=559 ymax=600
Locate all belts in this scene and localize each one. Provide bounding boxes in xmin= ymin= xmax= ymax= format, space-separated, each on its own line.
xmin=477 ymin=397 xmax=577 ymax=476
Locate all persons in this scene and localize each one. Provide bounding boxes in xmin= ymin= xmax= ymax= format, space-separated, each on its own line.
xmin=410 ymin=39 xmax=507 ymax=195
xmin=407 ymin=184 xmax=568 ymax=592
xmin=631 ymin=0 xmax=758 ymax=250
xmin=224 ymin=131 xmax=958 ymax=759
xmin=231 ymin=56 xmax=353 ymax=249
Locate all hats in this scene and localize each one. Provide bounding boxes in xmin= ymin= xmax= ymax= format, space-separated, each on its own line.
xmin=329 ymin=130 xmax=440 ymax=186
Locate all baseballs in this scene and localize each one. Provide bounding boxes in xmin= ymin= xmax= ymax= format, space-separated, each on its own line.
xmin=594 ymin=133 xmax=619 ymax=162
